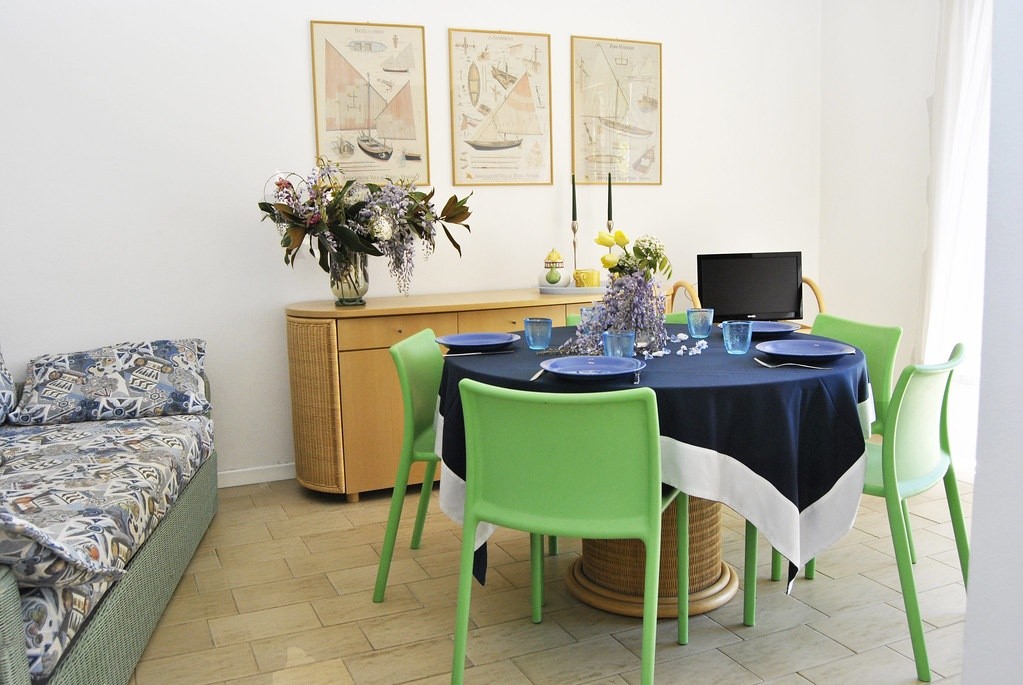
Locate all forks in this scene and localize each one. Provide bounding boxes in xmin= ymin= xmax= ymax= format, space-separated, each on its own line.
xmin=754 ymin=358 xmax=833 ymax=370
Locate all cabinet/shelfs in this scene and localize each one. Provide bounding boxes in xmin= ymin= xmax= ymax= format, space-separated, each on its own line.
xmin=286 ymin=293 xmax=609 ymax=501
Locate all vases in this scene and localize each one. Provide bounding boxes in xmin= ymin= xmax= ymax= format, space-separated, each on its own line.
xmin=328 ymin=245 xmax=369 ymax=305
xmin=632 ymin=328 xmax=650 ymax=348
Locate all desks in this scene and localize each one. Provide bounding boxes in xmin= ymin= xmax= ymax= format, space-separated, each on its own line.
xmin=436 ymin=323 xmax=874 ymax=629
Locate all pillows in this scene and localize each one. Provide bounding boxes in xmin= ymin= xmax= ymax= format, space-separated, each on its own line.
xmin=8 ymin=337 xmax=211 ymax=424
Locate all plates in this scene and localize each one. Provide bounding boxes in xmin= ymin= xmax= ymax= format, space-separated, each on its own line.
xmin=717 ymin=322 xmax=801 ymax=338
xmin=539 ymin=288 xmax=606 ymax=295
xmin=435 ymin=332 xmax=522 ymax=351
xmin=539 ymin=355 xmax=646 ymax=384
xmin=755 ymin=340 xmax=856 ymax=363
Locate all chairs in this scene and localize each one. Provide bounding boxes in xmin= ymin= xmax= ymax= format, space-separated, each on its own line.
xmin=802 ymin=346 xmax=965 ymax=682
xmin=808 ymin=314 xmax=901 ymax=440
xmin=371 ymin=328 xmax=554 ymax=624
xmin=447 ymin=378 xmax=689 ymax=685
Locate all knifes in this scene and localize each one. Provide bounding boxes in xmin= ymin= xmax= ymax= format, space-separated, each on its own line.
xmin=442 ymin=351 xmax=514 ymax=357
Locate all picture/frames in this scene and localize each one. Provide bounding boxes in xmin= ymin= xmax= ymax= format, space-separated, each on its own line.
xmin=570 ymin=34 xmax=662 ymax=185
xmin=447 ymin=28 xmax=554 ymax=188
xmin=309 ymin=20 xmax=430 ymax=187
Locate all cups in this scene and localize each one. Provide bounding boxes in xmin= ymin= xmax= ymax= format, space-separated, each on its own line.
xmin=722 ymin=320 xmax=753 ymax=354
xmin=580 ymin=306 xmax=606 ymax=334
xmin=687 ymin=308 xmax=714 ymax=339
xmin=603 ymin=330 xmax=635 ymax=358
xmin=524 ymin=318 xmax=552 ymax=350
xmin=573 ymin=269 xmax=601 ymax=287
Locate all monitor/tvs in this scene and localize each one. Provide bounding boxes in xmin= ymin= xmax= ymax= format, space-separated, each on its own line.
xmin=698 ymin=251 xmax=804 ymax=325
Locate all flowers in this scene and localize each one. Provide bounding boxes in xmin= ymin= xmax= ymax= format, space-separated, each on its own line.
xmin=552 ymin=232 xmax=675 ymax=353
xmin=258 ymin=154 xmax=474 ymax=295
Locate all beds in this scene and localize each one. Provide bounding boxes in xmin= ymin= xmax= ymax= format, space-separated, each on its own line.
xmin=0 ymin=373 xmax=218 ymax=685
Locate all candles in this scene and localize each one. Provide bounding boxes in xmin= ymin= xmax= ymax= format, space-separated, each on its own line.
xmin=608 ymin=173 xmax=612 ymax=220
xmin=572 ymin=174 xmax=576 ymax=220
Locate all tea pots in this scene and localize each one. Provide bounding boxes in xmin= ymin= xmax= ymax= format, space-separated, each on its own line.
xmin=538 ymin=247 xmax=570 ymax=287
